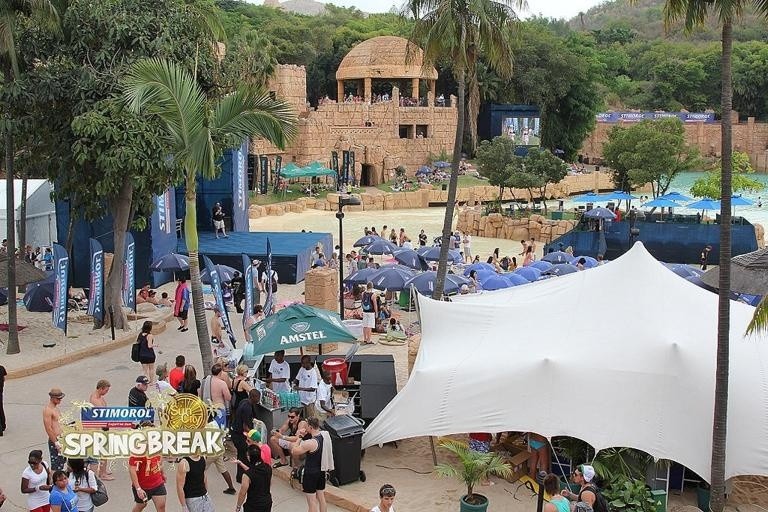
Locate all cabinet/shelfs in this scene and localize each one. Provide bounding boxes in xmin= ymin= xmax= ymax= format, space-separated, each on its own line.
xmin=253 ymin=400 xmax=291 ymax=460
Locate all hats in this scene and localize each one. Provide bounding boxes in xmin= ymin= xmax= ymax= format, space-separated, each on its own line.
xmin=136 ymin=376 xmax=151 ymax=385
xmin=49 ymin=388 xmax=65 ymax=399
xmin=242 ymin=428 xmax=260 ymax=442
xmin=156 ymin=364 xmax=167 ymax=380
xmin=253 ymin=260 xmax=261 ymax=267
xmin=580 ymin=465 xmax=594 ymax=481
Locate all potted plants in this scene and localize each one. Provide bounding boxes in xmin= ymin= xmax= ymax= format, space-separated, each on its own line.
xmin=433 ymin=435 xmax=514 ymax=512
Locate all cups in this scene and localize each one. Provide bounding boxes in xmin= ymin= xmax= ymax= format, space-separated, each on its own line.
xmin=142 ymin=495 xmax=148 ymax=506
xmin=321 ymin=357 xmax=348 ymax=386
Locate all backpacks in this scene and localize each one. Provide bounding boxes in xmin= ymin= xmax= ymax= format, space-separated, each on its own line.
xmin=264 ymin=270 xmax=277 ymax=293
xmin=362 ymin=292 xmax=371 ymax=311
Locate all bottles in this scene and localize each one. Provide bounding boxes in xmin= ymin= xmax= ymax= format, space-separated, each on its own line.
xmin=227 ymin=355 xmax=238 ymax=369
xmin=262 ymin=388 xmax=303 ymax=410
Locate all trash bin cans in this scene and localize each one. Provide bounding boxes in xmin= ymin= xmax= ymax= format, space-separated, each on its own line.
xmin=321 ymin=414 xmax=367 ymax=488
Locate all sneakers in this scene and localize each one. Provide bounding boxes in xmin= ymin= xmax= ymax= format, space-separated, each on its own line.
xmin=223 ymin=488 xmax=235 ymax=494
xmin=177 ymin=326 xmax=188 ymax=332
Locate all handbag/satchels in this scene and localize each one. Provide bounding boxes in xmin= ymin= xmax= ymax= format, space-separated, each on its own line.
xmin=131 ymin=344 xmax=140 ymax=363
xmin=590 ymin=486 xmax=609 ymax=511
xmin=91 ymin=480 xmax=108 ymax=506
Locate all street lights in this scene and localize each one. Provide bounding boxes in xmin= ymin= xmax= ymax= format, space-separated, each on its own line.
xmin=336 ymin=195 xmax=362 ymax=319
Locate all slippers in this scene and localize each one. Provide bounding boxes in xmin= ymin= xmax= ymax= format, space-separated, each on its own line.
xmin=168 ymin=457 xmax=180 ymax=463
xmin=364 ymin=340 xmax=374 ymax=345
xmin=273 ymin=462 xmax=288 ymax=468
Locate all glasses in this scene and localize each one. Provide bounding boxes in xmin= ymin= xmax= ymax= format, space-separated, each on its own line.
xmin=287 ymin=415 xmax=296 ymax=418
xmin=28 ymin=461 xmax=37 ymax=464
xmin=381 ymin=488 xmax=395 ymax=493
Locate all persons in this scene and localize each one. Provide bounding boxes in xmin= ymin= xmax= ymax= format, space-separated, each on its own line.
xmin=0 ymin=92 xmax=761 ymax=510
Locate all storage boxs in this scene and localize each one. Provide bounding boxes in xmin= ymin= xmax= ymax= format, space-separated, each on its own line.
xmin=492 ymin=444 xmax=532 ymax=484
